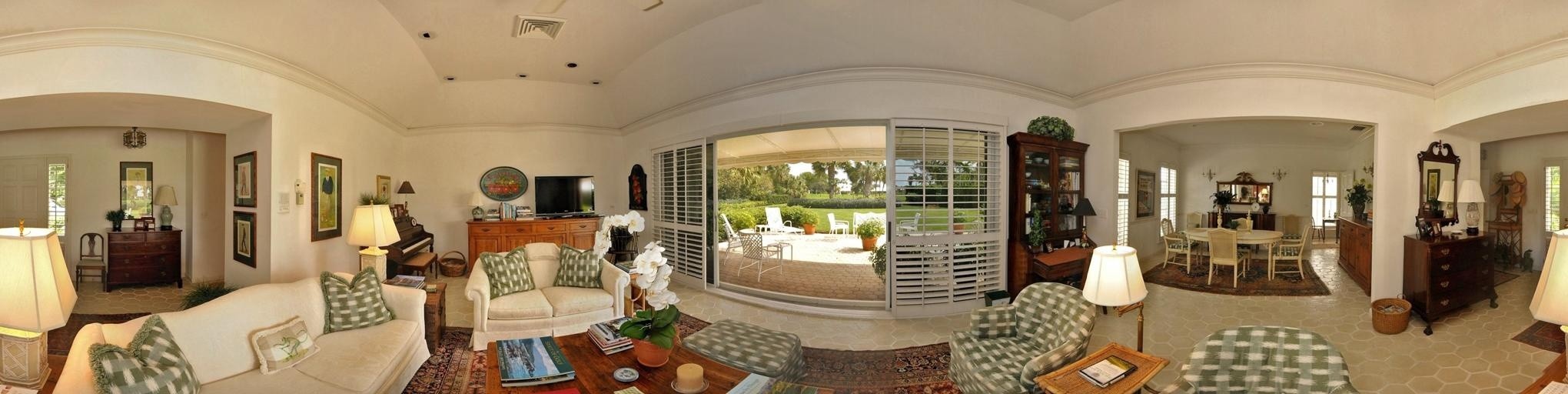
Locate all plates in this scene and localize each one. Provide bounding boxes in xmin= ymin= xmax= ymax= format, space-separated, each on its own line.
xmin=612 ymin=366 xmax=641 ymax=383
xmin=1451 ymin=230 xmax=1463 ymax=234
xmin=671 ymin=378 xmax=709 ymax=394
xmin=1251 ymin=202 xmax=1260 ymax=212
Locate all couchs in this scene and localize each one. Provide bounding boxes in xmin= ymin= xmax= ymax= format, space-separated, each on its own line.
xmin=51 ymin=270 xmax=427 ymax=394
xmin=466 ymin=240 xmax=630 ymax=351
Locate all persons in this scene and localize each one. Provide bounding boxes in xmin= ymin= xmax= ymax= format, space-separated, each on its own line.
xmin=241 ymin=223 xmax=247 ymax=252
xmin=320 ymin=168 xmax=333 ymax=222
xmin=241 ymin=166 xmax=247 ymax=195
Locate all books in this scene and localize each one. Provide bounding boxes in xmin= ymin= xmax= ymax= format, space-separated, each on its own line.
xmin=1025 ymin=217 xmax=1034 ymax=235
xmin=1058 ymin=193 xmax=1079 ymax=212
xmin=1026 ymin=193 xmax=1032 ymax=214
xmin=384 ymin=275 xmax=427 ymax=290
xmin=1060 ymin=156 xmax=1080 ymax=169
xmin=1076 ymin=355 xmax=1138 ymax=389
xmin=587 ymin=316 xmax=635 ymax=356
xmin=1058 ymin=214 xmax=1077 ymax=231
xmin=495 ymin=335 xmax=576 ymax=389
xmin=482 ymin=202 xmax=535 ymax=221
xmin=1063 ymin=172 xmax=1080 ymax=191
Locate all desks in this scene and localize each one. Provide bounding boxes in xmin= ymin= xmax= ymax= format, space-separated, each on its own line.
xmin=390 ymin=277 xmax=448 ymax=353
xmin=1033 ymin=340 xmax=1170 ymax=394
xmin=1515 ymin=350 xmax=1567 ymax=394
xmin=606 ymin=249 xmax=637 ymax=268
xmin=1322 ymin=216 xmax=1341 ymax=244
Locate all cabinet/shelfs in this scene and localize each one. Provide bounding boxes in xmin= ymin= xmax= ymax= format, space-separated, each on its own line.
xmin=1336 ymin=216 xmax=1373 ymax=296
xmin=106 ymin=227 xmax=183 ymax=293
xmin=1488 ymin=172 xmax=1526 ymax=271
xmin=1401 ymin=229 xmax=1498 ymax=334
xmin=1007 ymin=132 xmax=1096 ymax=300
xmin=463 ymin=217 xmax=600 ymax=276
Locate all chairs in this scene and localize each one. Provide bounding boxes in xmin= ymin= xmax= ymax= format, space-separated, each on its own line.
xmin=1158 ymin=322 xmax=1359 ymax=394
xmin=826 ymin=212 xmax=850 ymax=240
xmin=948 ymin=282 xmax=1098 ymax=394
xmin=1311 ymin=216 xmax=1325 ymax=242
xmin=898 ymin=212 xmax=922 ymax=236
xmin=721 ymin=206 xmax=805 ymax=281
xmin=1158 ymin=212 xmax=1312 ymax=289
xmin=75 ymin=233 xmax=107 ymax=293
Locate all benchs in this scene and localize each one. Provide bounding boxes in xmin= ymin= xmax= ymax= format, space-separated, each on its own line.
xmin=851 ymin=209 xmax=887 ymax=235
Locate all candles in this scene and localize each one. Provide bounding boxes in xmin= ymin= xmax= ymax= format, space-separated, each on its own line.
xmin=677 ymin=362 xmax=704 ymax=392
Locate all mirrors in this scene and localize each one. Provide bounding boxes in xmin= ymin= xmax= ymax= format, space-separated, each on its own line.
xmin=1416 ymin=138 xmax=1460 ymax=226
xmin=1216 ymin=171 xmax=1273 ymax=206
xmin=1502 ymin=183 xmax=1517 ymax=210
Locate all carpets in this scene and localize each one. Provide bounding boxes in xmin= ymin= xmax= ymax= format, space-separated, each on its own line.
xmin=1512 ymin=319 xmax=1566 ymax=354
xmin=49 ymin=311 xmax=152 ymax=357
xmin=402 ymin=308 xmax=958 ymax=393
xmin=1140 ymin=253 xmax=1333 ymax=299
xmin=1492 ymin=268 xmax=1521 ymax=287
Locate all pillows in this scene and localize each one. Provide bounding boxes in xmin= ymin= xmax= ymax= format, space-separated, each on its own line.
xmin=252 ymin=316 xmax=320 ymax=375
xmin=89 ymin=311 xmax=203 ymax=394
xmin=554 ymin=241 xmax=605 ymax=290
xmin=320 ymin=267 xmax=394 ymax=336
xmin=480 ymin=247 xmax=536 ymax=300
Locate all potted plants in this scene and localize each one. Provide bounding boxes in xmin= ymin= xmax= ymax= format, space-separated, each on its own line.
xmin=856 ymin=217 xmax=885 ymax=252
xmin=949 ymin=211 xmax=971 ymax=235
xmin=1210 ymin=190 xmax=1238 ymax=213
xmin=106 ymin=209 xmax=126 ymax=231
xmin=799 ymin=211 xmax=819 ymax=235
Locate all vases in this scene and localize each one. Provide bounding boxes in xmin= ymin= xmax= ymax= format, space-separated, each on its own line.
xmin=1351 ymin=203 xmax=1365 ymax=219
xmin=610 ymin=225 xmax=635 ymax=252
xmin=629 ymin=334 xmax=676 ymax=368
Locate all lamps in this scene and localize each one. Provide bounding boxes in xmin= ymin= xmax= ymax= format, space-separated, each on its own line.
xmin=1456 ymin=178 xmax=1485 ymax=232
xmin=1362 ymin=160 xmax=1373 ymax=177
xmin=1201 ymin=165 xmax=1217 ymax=182
xmin=1086 ymin=241 xmax=1148 ymax=392
xmin=1070 ymin=197 xmax=1096 ymax=230
xmin=0 ymin=215 xmax=78 ymax=394
xmin=347 ymin=198 xmax=401 ymax=285
xmin=122 ymin=125 xmax=147 ymax=148
xmin=1436 ymin=178 xmax=1454 ymax=219
xmin=1270 ymin=165 xmax=1288 ymax=181
xmin=398 ymin=179 xmax=416 ymax=210
xmin=154 ymin=185 xmax=178 ymax=230
xmin=1530 ymin=228 xmax=1567 ymax=383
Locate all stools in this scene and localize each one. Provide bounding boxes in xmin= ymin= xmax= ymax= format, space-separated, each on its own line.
xmin=402 ymin=251 xmax=439 ymax=281
xmin=685 ymin=317 xmax=809 ymax=384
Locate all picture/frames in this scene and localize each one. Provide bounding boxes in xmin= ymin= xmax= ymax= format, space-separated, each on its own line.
xmin=628 ymin=163 xmax=648 ymax=211
xmin=311 ymin=152 xmax=342 ymax=243
xmin=233 ymin=150 xmax=257 ymax=208
xmin=1426 ymin=168 xmax=1440 ymax=204
xmin=1136 ymin=167 xmax=1156 ymax=221
xmin=231 ymin=210 xmax=256 ymax=268
xmin=376 ymin=174 xmax=391 ymax=204
xmin=479 ymin=165 xmax=527 ymax=202
xmin=134 ymin=217 xmax=156 ymax=231
xmin=119 ymin=161 xmax=153 ymax=221
xmin=1415 ymin=216 xmax=1442 ymax=237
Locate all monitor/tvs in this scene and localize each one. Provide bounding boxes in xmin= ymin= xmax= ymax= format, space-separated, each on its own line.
xmin=533 ymin=174 xmax=598 ymax=218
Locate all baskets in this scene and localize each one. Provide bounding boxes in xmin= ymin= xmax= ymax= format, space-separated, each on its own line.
xmin=436 ymin=250 xmax=467 ymax=277
xmin=1370 ymin=293 xmax=1414 ymax=335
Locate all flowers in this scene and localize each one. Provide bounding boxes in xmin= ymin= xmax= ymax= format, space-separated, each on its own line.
xmin=621 ymin=236 xmax=680 ymax=349
xmin=1343 ymin=177 xmax=1373 ymax=205
xmin=593 ymin=209 xmax=644 ymax=259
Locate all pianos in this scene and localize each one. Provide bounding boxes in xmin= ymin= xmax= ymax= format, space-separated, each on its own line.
xmin=361 ymin=216 xmax=434 ymax=276
